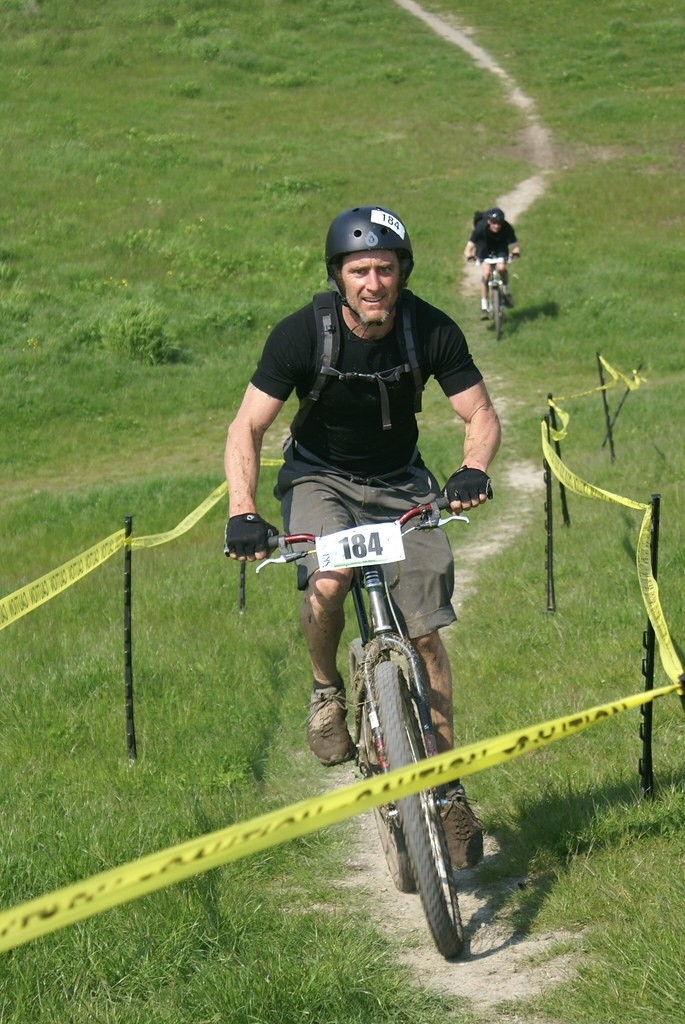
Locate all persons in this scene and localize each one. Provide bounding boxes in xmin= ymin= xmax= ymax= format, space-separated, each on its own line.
xmin=224 ymin=206 xmax=502 ymax=871
xmin=464 ymin=208 xmax=521 ymax=321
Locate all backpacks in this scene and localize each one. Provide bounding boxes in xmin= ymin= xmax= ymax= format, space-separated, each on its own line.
xmin=473 ymin=212 xmax=486 ymax=227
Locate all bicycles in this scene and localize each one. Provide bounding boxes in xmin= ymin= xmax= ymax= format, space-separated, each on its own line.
xmin=255 ymin=490 xmax=466 ymax=960
xmin=472 ymin=255 xmax=513 ymax=339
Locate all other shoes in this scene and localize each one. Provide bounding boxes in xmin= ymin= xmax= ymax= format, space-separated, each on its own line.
xmin=503 ymin=293 xmax=514 ymax=308
xmin=479 ymin=309 xmax=490 ymax=321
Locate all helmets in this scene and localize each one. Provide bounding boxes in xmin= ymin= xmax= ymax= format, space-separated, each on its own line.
xmin=489 ymin=208 xmax=505 ymax=225
xmin=326 ymin=206 xmax=415 ymax=283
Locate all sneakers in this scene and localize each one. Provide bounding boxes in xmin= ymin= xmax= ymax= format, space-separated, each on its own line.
xmin=438 ymin=784 xmax=484 ymax=871
xmin=304 ymin=684 xmax=357 ymax=767
